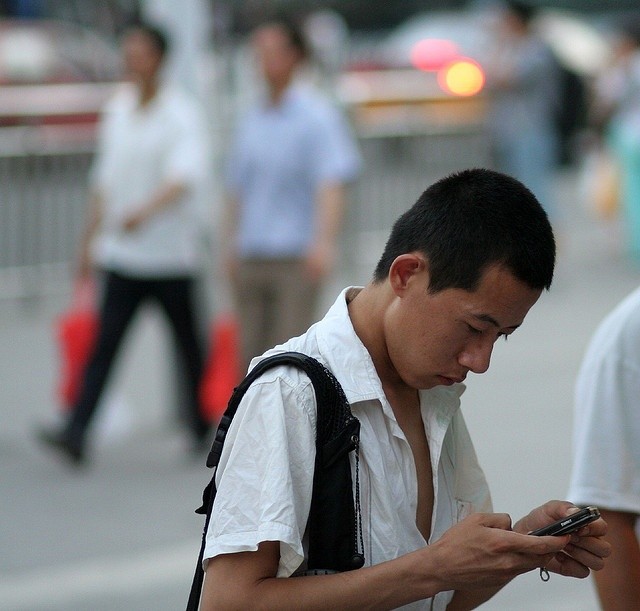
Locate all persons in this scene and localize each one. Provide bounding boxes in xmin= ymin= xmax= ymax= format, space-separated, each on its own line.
xmin=197 ymin=169 xmax=612 ymax=611
xmin=566 ymin=285 xmax=638 ymax=610
xmin=35 ymin=18 xmax=218 ymax=468
xmin=473 ymin=2 xmax=578 ymax=238
xmin=219 ymin=19 xmax=361 ymax=377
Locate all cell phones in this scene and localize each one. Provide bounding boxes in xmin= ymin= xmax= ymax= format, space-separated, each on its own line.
xmin=526 ymin=503 xmax=600 ymax=537
